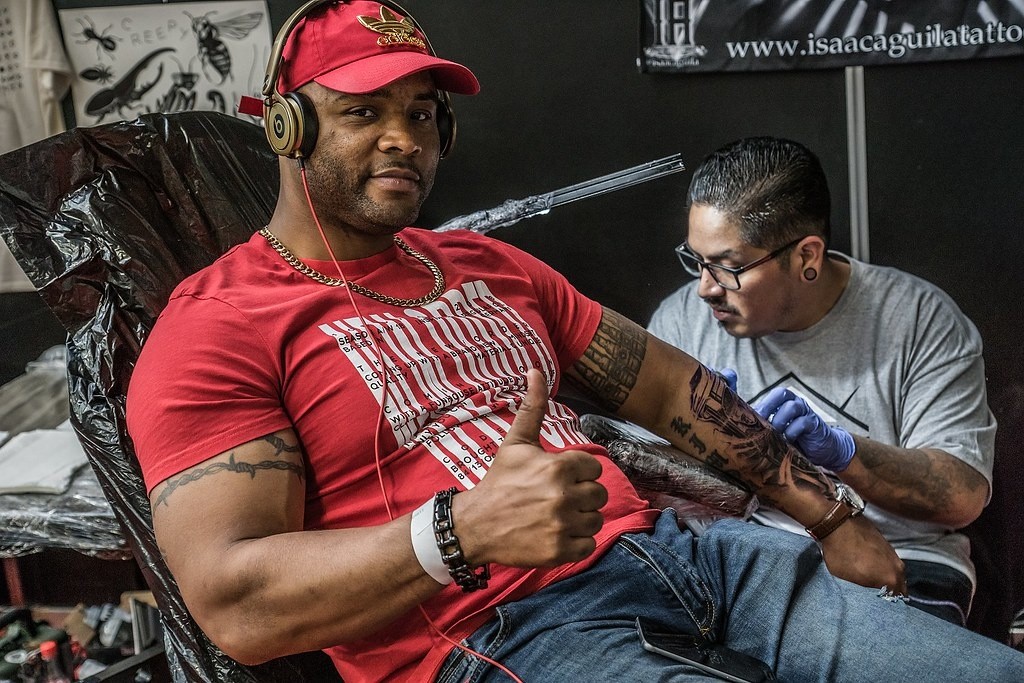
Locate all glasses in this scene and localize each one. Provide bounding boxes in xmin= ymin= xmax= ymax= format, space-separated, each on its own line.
xmin=673 ymin=236 xmax=803 ymax=293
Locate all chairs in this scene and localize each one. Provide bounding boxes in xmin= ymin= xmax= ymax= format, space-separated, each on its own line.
xmin=0 ymin=111 xmax=764 ymax=683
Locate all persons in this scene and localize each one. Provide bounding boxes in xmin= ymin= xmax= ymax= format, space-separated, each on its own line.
xmin=121 ymin=0 xmax=1023 ymax=683
xmin=620 ymin=135 xmax=997 ymax=626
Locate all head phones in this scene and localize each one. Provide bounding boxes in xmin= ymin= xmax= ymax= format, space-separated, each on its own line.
xmin=264 ymin=0 xmax=456 ymax=161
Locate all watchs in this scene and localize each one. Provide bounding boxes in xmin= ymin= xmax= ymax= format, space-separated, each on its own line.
xmin=804 ymin=481 xmax=865 ymax=540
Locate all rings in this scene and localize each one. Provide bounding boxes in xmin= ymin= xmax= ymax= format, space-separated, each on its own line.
xmin=899 ymin=579 xmax=908 ymax=584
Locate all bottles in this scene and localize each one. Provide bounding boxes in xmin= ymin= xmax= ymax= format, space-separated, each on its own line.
xmin=40 ymin=641 xmax=75 ymax=683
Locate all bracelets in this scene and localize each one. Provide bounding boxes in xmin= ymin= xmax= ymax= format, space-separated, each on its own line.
xmin=433 ymin=485 xmax=492 ymax=592
xmin=408 ymin=496 xmax=456 ymax=586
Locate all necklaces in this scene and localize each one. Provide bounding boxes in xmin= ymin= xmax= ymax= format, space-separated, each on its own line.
xmin=256 ymin=221 xmax=445 ymax=308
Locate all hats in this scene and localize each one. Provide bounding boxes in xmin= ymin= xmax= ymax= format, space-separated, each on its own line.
xmin=276 ymin=0 xmax=481 ymax=96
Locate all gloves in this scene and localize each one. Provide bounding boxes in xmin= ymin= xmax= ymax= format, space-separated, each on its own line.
xmin=754 ymin=387 xmax=856 ymax=474
xmin=705 ymin=366 xmax=738 ymax=394
